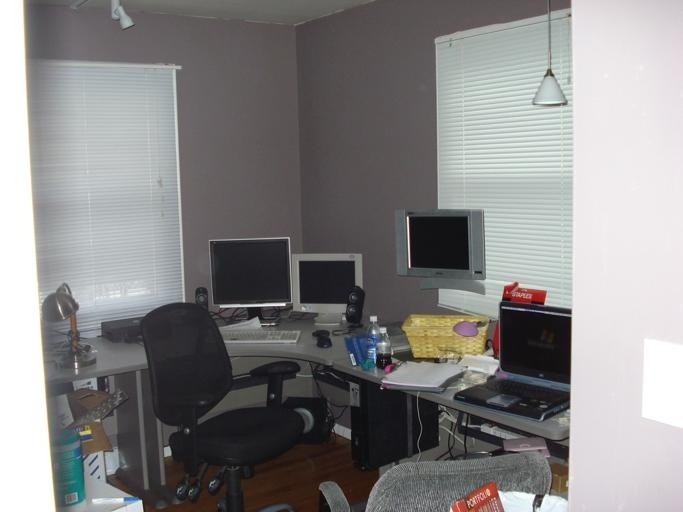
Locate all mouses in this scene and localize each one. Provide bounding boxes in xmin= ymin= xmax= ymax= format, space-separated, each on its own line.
xmin=318 ymin=336 xmax=332 ymax=348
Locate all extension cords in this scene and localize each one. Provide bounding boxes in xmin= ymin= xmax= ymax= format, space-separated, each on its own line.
xmin=480 ymin=423 xmax=527 ymax=439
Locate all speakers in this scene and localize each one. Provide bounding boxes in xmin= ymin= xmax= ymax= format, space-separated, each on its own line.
xmin=196 ymin=287 xmax=208 ymax=308
xmin=284 ymin=397 xmax=328 ymax=444
xmin=345 ymin=286 xmax=365 ymax=327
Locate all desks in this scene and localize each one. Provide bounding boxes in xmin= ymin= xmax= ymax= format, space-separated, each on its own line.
xmin=43 ymin=311 xmax=569 ymax=509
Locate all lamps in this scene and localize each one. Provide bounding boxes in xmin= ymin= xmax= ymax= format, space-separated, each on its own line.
xmin=532 ymin=0 xmax=569 ymax=105
xmin=41 ymin=283 xmax=97 ymax=369
xmin=110 ymin=0 xmax=135 ymax=31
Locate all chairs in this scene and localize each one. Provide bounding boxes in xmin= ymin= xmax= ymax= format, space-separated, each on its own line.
xmin=314 ymin=451 xmax=553 ymax=512
xmin=140 ymin=302 xmax=316 ymax=512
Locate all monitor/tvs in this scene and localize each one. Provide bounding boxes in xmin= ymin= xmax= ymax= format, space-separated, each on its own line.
xmin=292 ymin=253 xmax=364 ymax=326
xmin=394 ymin=208 xmax=486 ymax=281
xmin=208 ymin=237 xmax=291 ymax=326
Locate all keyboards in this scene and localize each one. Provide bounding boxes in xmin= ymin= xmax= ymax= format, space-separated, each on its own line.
xmin=221 ymin=330 xmax=301 ymax=344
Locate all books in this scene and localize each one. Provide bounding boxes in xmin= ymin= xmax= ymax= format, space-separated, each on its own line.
xmin=449 ymin=480 xmax=506 ymax=512
xmin=382 ymin=359 xmax=468 ymax=393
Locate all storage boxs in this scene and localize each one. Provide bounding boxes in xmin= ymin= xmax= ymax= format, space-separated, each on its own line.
xmin=48 ymin=386 xmax=120 ymax=484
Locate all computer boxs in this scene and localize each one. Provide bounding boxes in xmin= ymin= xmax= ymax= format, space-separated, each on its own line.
xmin=351 ymin=376 xmax=440 ymax=470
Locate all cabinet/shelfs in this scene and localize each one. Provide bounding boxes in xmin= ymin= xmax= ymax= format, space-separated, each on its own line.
xmin=55 ymin=471 xmax=144 ymax=512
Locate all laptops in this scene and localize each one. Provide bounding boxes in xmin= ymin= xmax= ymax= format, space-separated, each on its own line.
xmin=454 ymin=301 xmax=571 ymax=422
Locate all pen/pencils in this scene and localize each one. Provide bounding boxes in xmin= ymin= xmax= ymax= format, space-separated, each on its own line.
xmin=91 ymin=497 xmax=139 ymax=504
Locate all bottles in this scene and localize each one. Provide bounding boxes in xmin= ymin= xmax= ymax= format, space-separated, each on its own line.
xmin=366 ymin=316 xmax=379 ymax=360
xmin=375 ymin=325 xmax=392 ymax=369
